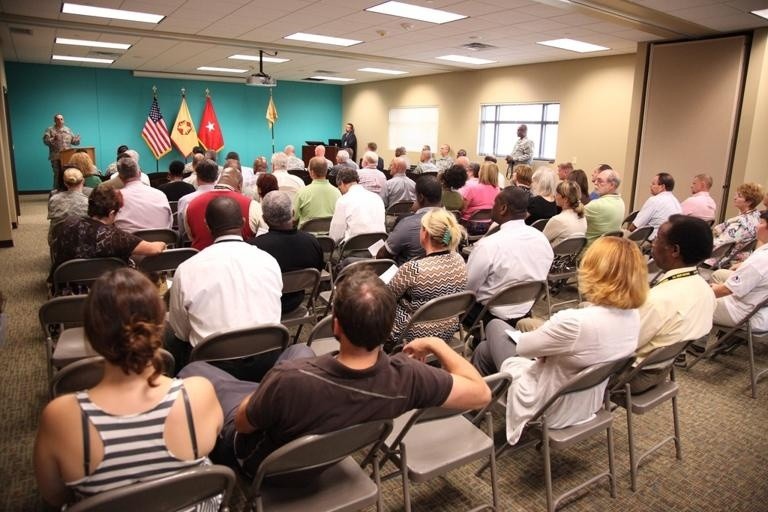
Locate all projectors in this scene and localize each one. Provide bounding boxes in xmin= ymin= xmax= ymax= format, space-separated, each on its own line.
xmin=245 ymin=75 xmax=276 ymax=87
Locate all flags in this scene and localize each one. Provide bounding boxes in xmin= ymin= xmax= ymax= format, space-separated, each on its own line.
xmin=264 ymin=95 xmax=279 ymax=129
xmin=140 ymin=96 xmax=173 ymax=159
xmin=168 ymin=95 xmax=199 ymax=160
xmin=196 ymin=95 xmax=224 ymax=154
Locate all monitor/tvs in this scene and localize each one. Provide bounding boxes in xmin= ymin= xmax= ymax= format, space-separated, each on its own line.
xmin=329 ymin=139 xmax=342 ymax=148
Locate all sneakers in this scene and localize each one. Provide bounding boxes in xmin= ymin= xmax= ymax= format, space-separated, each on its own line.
xmin=673 ymin=353 xmax=687 ymax=368
xmin=686 ymin=343 xmax=705 ymax=356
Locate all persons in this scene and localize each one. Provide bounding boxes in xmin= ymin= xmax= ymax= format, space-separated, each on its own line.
xmin=43 ymin=113 xmax=81 ymax=194
xmin=340 ymin=122 xmax=357 ymax=162
xmin=30 ymin=141 xmax=767 ymax=512
xmin=505 ymin=124 xmax=535 ymax=183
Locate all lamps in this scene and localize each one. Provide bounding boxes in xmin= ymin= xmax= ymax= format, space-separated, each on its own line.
xmin=245 ymin=49 xmax=279 ymax=87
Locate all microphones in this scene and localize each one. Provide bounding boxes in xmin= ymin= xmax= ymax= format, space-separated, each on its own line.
xmin=63 ymin=130 xmax=70 ymax=135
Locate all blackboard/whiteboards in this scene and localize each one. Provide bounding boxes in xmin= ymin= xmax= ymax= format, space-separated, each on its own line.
xmin=388 ymin=105 xmax=440 ymax=154
